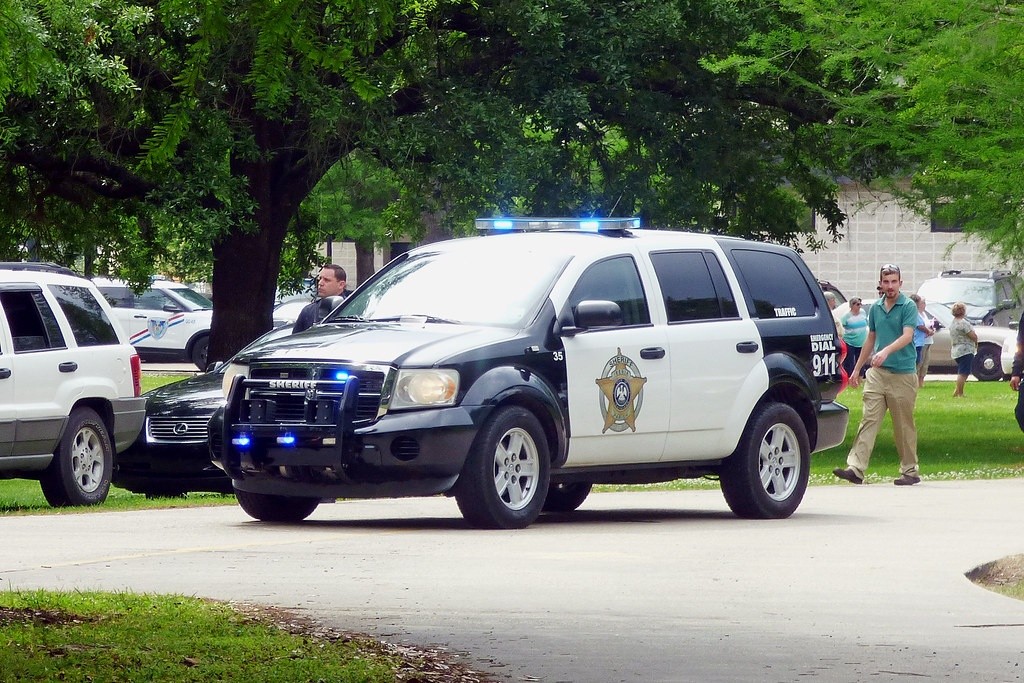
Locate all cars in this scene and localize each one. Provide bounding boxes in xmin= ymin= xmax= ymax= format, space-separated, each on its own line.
xmin=272 ymin=277 xmax=323 ymax=328
xmin=110 ymin=323 xmax=297 ymax=499
xmin=831 ymin=297 xmax=1019 ymax=382
xmin=815 ymin=280 xmax=848 ymax=309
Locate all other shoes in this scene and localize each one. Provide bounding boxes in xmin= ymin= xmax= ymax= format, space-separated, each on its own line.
xmin=833 ymin=467 xmax=862 ymax=484
xmin=894 ymin=475 xmax=920 ymax=485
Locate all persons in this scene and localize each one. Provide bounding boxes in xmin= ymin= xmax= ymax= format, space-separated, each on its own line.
xmin=823 ymin=285 xmax=935 ymax=387
xmin=292 ymin=265 xmax=354 ymax=334
xmin=949 ymin=302 xmax=978 ymax=397
xmin=833 ymin=263 xmax=920 ymax=485
xmin=1010 ymin=310 xmax=1024 ymax=433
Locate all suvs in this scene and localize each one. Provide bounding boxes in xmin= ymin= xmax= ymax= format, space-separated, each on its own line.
xmin=908 ymin=269 xmax=1024 ymax=325
xmin=89 ymin=273 xmax=214 ymax=372
xmin=1 ymin=260 xmax=146 ymax=509
xmin=205 ymin=215 xmax=851 ymax=529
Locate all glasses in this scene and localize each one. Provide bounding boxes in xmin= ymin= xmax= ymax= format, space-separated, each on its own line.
xmin=881 ymin=264 xmax=899 ymax=271
xmin=857 ymin=303 xmax=862 ymax=306
